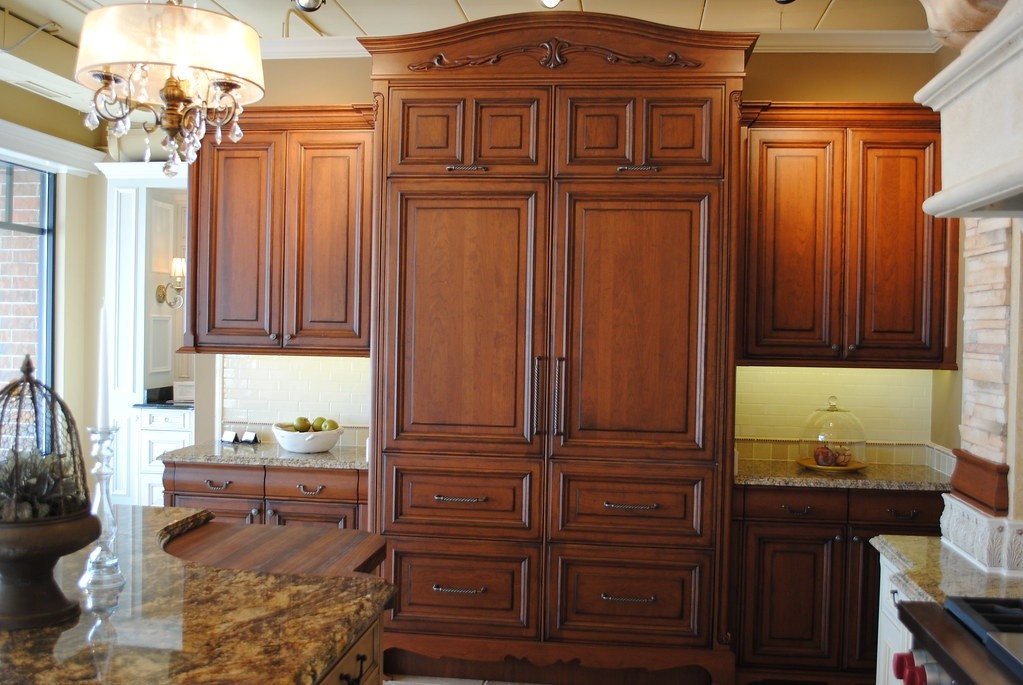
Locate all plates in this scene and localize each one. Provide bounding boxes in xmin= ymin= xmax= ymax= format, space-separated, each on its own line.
xmin=797 ymin=458 xmax=866 ymax=473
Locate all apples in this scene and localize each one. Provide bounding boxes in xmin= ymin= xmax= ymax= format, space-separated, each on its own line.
xmin=294 ymin=416 xmax=339 ymax=432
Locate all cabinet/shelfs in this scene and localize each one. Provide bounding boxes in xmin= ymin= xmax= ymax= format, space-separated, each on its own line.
xmin=0 ymin=11 xmax=958 ymax=685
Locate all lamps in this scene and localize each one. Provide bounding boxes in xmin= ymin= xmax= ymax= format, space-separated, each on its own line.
xmin=156 ymin=257 xmax=186 ymax=309
xmin=75 ymin=0 xmax=264 ymax=176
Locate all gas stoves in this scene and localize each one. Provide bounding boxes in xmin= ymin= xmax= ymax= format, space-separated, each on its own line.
xmin=942 ymin=595 xmax=1023 ymax=678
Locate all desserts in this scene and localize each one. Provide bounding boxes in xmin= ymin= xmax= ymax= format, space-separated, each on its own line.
xmin=816 ymin=444 xmax=851 ymax=465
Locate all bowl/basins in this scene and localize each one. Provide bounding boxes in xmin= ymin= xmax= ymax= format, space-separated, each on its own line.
xmin=272 ymin=422 xmax=342 ymax=452
